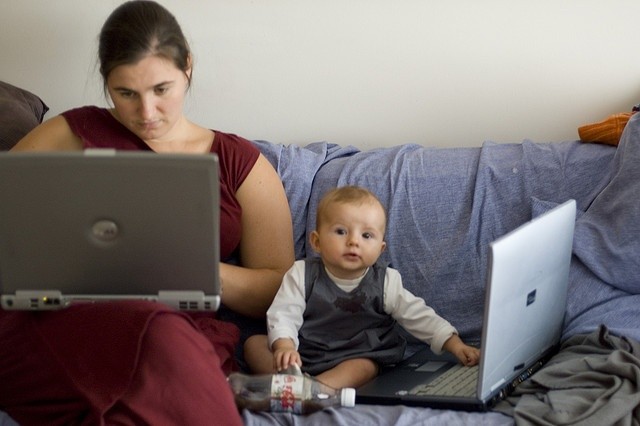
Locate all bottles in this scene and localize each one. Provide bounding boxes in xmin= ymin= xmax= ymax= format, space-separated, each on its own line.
xmin=225 ymin=372 xmax=357 ymax=415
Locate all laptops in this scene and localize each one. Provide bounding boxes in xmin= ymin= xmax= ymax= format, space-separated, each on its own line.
xmin=357 ymin=199 xmax=575 ymax=414
xmin=0 ymin=154 xmax=220 ymax=312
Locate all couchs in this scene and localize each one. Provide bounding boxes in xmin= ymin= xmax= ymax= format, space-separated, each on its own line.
xmin=243 ymin=137 xmax=639 ymax=426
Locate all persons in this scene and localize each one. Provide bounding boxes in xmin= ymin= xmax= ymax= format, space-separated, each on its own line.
xmin=243 ymin=185 xmax=480 ymax=393
xmin=4 ymin=1 xmax=296 ymax=426
